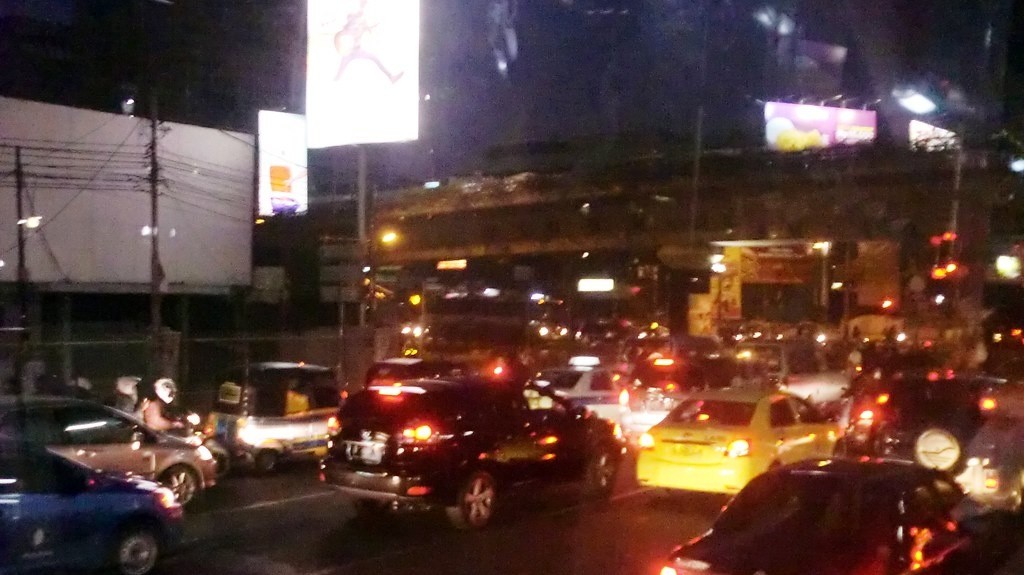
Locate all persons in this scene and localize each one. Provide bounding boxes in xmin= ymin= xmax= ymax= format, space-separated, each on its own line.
xmin=112 ymin=375 xmax=192 ymax=433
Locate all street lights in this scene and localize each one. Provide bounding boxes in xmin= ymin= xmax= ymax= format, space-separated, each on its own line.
xmin=355 ymin=227 xmax=398 ymax=323
xmin=15 ymin=214 xmax=44 ymax=343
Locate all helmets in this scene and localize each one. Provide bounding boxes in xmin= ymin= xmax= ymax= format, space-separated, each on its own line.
xmin=154 ymin=378 xmax=176 ymax=404
xmin=117 ymin=377 xmax=142 ymax=396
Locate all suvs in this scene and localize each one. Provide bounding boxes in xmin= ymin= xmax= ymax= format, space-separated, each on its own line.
xmin=320 ymin=375 xmax=626 ymax=535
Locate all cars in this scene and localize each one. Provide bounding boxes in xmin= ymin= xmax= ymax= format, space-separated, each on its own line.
xmin=631 ymin=385 xmax=847 ymax=497
xmin=204 ymin=360 xmax=342 ymax=476
xmin=0 ymin=427 xmax=184 ymax=575
xmin=655 ymin=455 xmax=1005 ymax=575
xmin=520 ymin=319 xmax=985 ymax=455
xmin=0 ymin=391 xmax=221 ymax=508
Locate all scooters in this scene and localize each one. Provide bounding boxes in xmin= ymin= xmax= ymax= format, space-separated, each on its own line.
xmin=173 ymin=409 xmax=230 ymax=480
